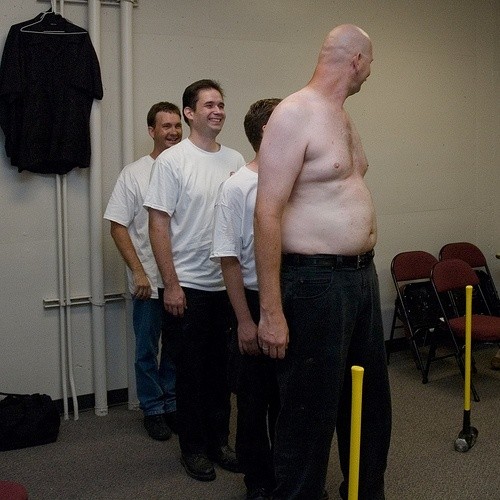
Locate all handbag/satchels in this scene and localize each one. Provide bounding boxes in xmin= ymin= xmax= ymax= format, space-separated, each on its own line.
xmin=0 ymin=394 xmax=61 ymax=451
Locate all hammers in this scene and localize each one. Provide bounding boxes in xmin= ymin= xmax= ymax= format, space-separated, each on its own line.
xmin=347 ymin=364 xmax=364 ymax=500
xmin=454 ymin=286 xmax=479 ymax=452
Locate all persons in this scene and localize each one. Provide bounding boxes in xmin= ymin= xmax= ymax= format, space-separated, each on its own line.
xmin=143 ymin=79 xmax=246 ymax=482
xmin=210 ymin=99 xmax=283 ymax=500
xmin=104 ymin=103 xmax=183 ymax=442
xmin=254 ymin=24 xmax=393 ymax=500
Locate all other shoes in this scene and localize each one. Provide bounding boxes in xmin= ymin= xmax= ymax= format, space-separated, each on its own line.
xmin=144 ymin=412 xmax=179 ymax=442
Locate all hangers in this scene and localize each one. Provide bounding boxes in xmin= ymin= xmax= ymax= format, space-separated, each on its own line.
xmin=20 ymin=0 xmax=90 ymax=35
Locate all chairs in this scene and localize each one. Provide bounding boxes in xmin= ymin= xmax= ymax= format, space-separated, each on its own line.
xmin=389 ymin=242 xmax=500 ymax=401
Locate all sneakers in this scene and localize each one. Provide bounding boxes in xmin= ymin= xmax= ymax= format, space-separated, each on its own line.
xmin=180 ymin=442 xmax=216 ymax=482
xmin=207 ymin=444 xmax=243 ymax=473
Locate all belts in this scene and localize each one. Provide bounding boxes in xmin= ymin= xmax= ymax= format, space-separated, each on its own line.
xmin=285 ymin=250 xmax=375 ymax=267
